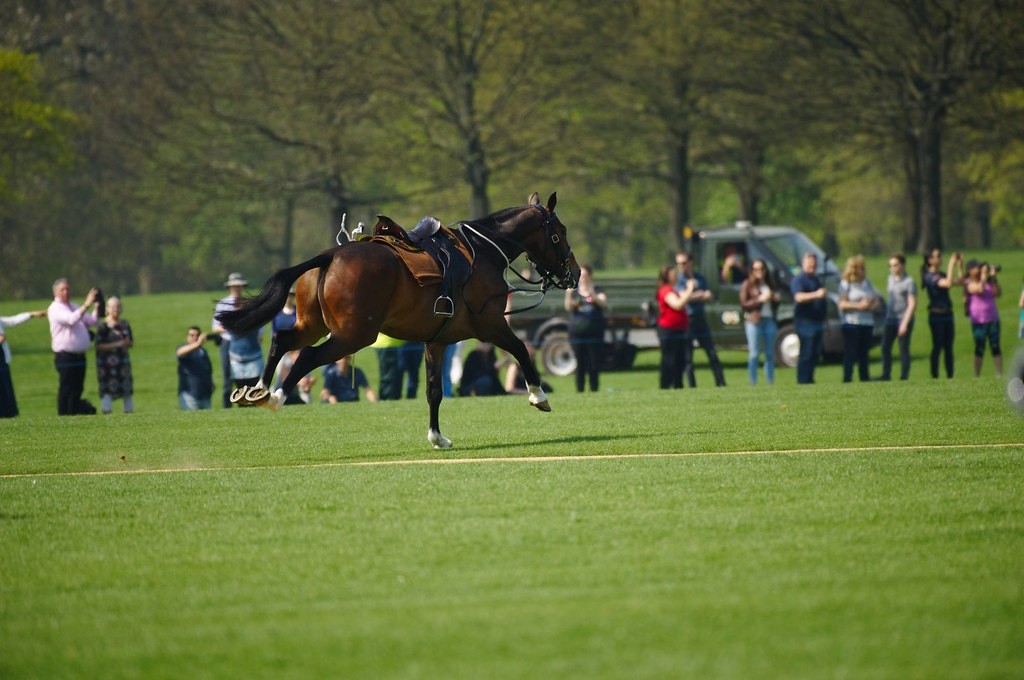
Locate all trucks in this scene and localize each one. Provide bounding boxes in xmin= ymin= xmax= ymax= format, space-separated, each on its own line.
xmin=503 ymin=220 xmax=889 ymax=371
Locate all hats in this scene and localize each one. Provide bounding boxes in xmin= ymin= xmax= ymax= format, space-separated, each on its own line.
xmin=965 ymin=259 xmax=982 ymax=271
xmin=224 ymin=273 xmax=246 ymax=287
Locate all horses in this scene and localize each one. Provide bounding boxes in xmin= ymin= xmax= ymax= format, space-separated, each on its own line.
xmin=213 ymin=191 xmax=581 ymax=449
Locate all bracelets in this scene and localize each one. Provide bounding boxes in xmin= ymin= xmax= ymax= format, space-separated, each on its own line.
xmin=32 ymin=312 xmax=35 ymax=317
xmin=83 ymin=304 xmax=89 ymax=309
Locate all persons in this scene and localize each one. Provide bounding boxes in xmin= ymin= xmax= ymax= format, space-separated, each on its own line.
xmin=1018 ymin=290 xmax=1024 ymax=340
xmin=565 ymin=265 xmax=608 ymax=392
xmin=790 ymin=252 xmax=827 ymax=384
xmin=720 ymin=245 xmax=747 ymax=284
xmin=48 ymin=278 xmax=99 ymax=415
xmin=0 ymin=309 xmax=46 ymax=418
xmin=739 ymin=260 xmax=781 ymax=385
xmin=94 ymin=297 xmax=133 ymax=413
xmin=372 ymin=334 xmax=423 ymax=399
xmin=212 ymin=272 xmax=316 ymax=408
xmin=837 ymin=257 xmax=879 ymax=382
xmin=176 ymin=327 xmax=215 ymax=411
xmin=656 ymin=251 xmax=726 ymax=388
xmin=321 ymin=355 xmax=376 ymax=403
xmin=920 ymin=249 xmax=965 ymax=378
xmin=962 ymin=260 xmax=1003 ymax=377
xmin=442 ymin=341 xmax=553 ymax=398
xmin=875 ymin=256 xmax=916 ymax=382
